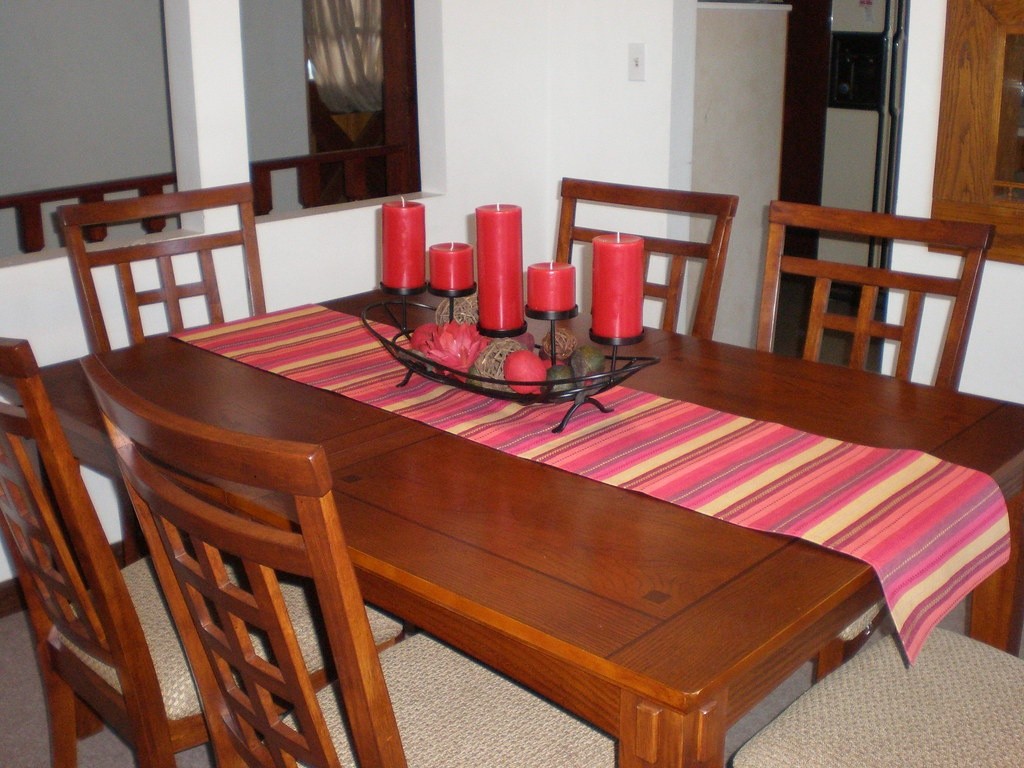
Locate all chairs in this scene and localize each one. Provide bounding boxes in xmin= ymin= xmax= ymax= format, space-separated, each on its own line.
xmin=78 ymin=353 xmax=618 ymax=768
xmin=56 ymin=183 xmax=268 ymax=570
xmin=1 ymin=336 xmax=405 ymax=767
xmin=753 ymin=201 xmax=996 ymax=682
xmin=554 ymin=174 xmax=740 ymax=350
xmin=726 ymin=621 xmax=1024 ymax=768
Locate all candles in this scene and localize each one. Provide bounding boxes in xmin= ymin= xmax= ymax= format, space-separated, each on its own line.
xmin=428 ymin=242 xmax=474 ymax=291
xmin=591 ymin=231 xmax=643 ymax=338
xmin=527 ymin=261 xmax=576 ymax=312
xmin=475 ymin=201 xmax=524 ymax=331
xmin=382 ymin=196 xmax=425 ymax=289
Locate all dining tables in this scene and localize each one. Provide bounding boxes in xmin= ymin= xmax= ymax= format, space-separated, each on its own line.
xmin=0 ymin=283 xmax=1023 ymax=768
